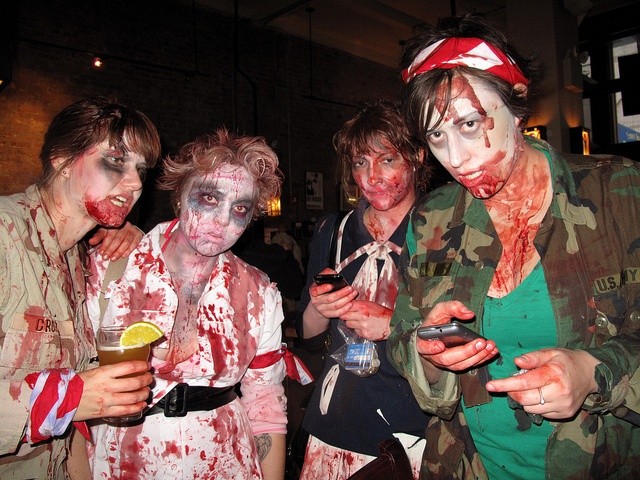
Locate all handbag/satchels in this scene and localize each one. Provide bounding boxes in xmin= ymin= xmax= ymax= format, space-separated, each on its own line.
xmin=288 ymin=209 xmax=349 ymax=465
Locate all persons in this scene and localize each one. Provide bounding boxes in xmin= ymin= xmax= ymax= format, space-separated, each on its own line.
xmin=303 ymin=215 xmax=318 ymax=232
xmin=291 ymin=98 xmax=437 ymax=479
xmin=66 ymin=127 xmax=314 ymax=480
xmin=228 ymin=219 xmax=305 ymax=318
xmin=386 ymin=9 xmax=639 ymax=479
xmin=270 ymin=233 xmax=305 ymax=280
xmin=0 ymin=95 xmax=161 ymax=479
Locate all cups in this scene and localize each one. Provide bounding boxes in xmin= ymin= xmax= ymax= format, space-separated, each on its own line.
xmin=97 ymin=326 xmax=150 ymax=426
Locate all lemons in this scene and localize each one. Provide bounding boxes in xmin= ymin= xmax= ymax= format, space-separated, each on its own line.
xmin=114 ymin=321 xmax=164 ymax=351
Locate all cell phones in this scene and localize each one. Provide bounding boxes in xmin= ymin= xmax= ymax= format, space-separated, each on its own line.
xmin=417 ymin=320 xmax=489 ymax=349
xmin=313 ymin=273 xmax=349 ymax=292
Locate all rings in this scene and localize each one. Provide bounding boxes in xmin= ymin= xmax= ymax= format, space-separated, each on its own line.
xmin=538 ymin=388 xmax=545 ymax=404
